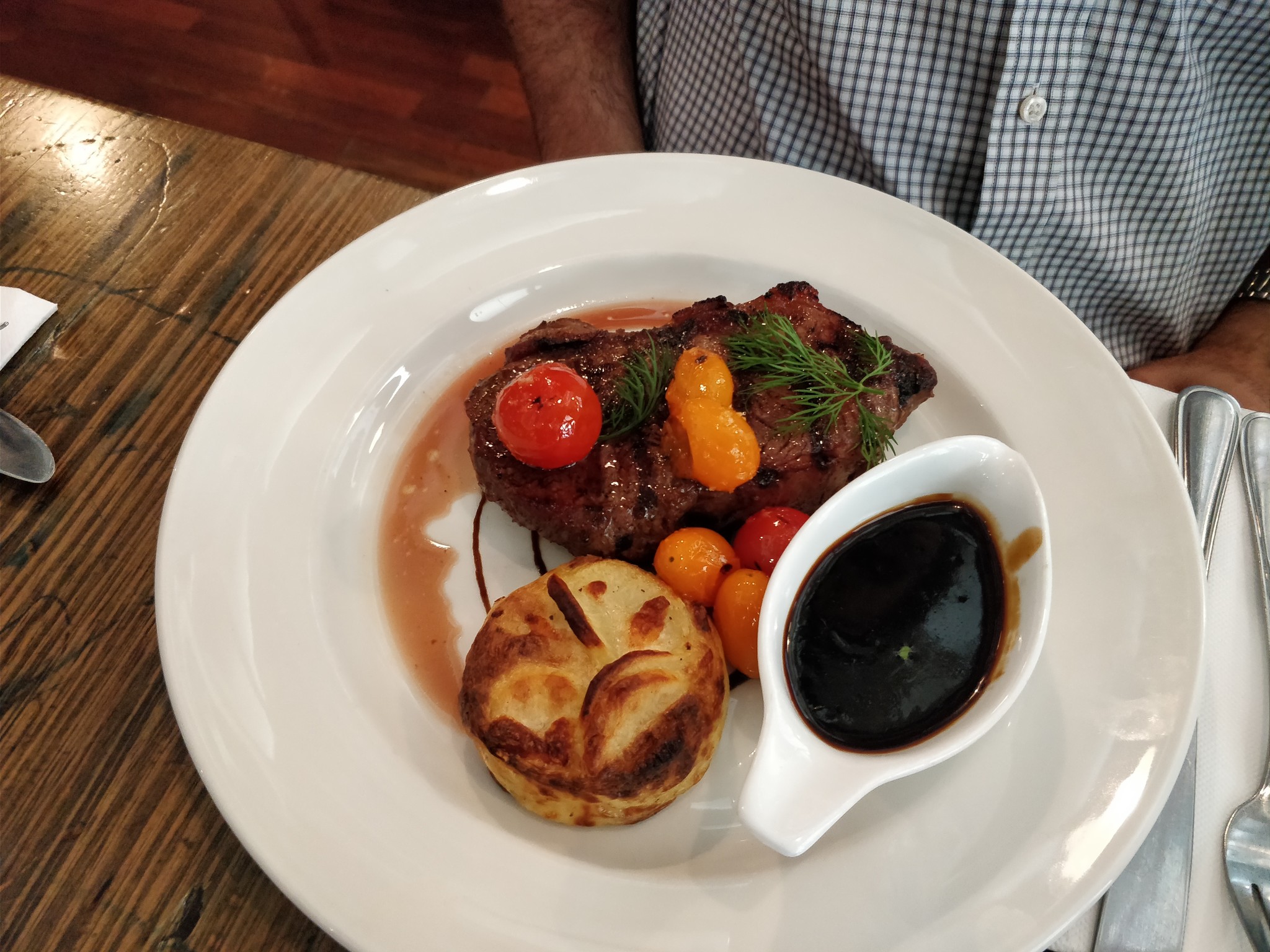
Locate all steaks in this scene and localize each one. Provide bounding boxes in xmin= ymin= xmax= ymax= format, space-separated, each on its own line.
xmin=463 ymin=281 xmax=941 ymax=563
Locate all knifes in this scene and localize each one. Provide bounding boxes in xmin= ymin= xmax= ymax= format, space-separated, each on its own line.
xmin=1092 ymin=386 xmax=1245 ymax=952
xmin=0 ymin=411 xmax=58 ymax=487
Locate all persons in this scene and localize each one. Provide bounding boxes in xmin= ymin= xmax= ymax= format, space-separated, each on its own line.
xmin=498 ymin=0 xmax=1270 ymax=369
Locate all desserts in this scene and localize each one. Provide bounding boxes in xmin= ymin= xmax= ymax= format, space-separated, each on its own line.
xmin=457 ymin=555 xmax=734 ymax=829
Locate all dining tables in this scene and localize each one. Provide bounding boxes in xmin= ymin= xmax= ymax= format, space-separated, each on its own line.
xmin=0 ymin=76 xmax=1270 ymax=952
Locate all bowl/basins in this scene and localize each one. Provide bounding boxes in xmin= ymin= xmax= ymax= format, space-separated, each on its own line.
xmin=740 ymin=433 xmax=1053 ymax=858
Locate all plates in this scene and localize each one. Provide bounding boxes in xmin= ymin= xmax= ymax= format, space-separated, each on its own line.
xmin=152 ymin=150 xmax=1211 ymax=949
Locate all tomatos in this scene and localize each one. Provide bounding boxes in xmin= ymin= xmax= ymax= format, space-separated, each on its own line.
xmin=491 ymin=363 xmax=600 ymax=468
xmin=666 ymin=342 xmax=757 ymax=492
xmin=651 ymin=505 xmax=812 ymax=680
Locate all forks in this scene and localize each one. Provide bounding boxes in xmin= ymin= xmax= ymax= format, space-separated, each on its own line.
xmin=1217 ymin=407 xmax=1269 ymax=952
